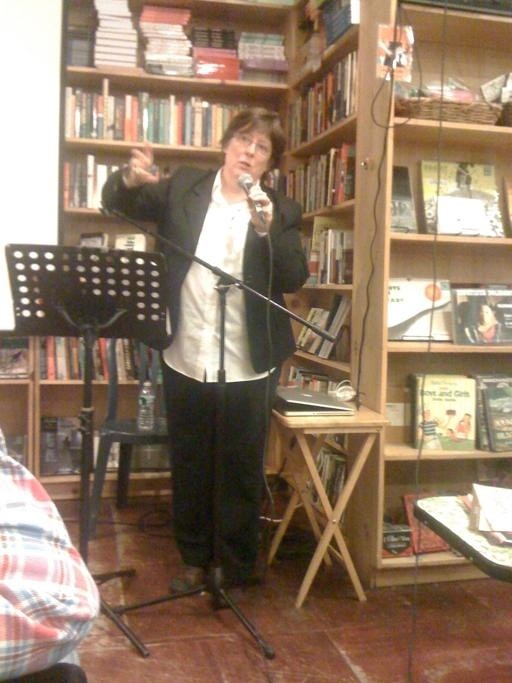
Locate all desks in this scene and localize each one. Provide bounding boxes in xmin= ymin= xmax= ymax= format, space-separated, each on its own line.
xmin=269 ymin=401 xmax=391 ymax=607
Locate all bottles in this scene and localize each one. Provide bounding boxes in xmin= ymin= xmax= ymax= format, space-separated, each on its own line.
xmin=138 ymin=382 xmax=155 ymax=430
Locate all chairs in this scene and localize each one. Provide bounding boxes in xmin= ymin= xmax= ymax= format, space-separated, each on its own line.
xmin=93 ymin=337 xmax=173 ymax=509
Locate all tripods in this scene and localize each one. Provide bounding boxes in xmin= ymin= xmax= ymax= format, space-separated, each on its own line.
xmin=114 ymin=210 xmax=278 ymax=662
xmin=69 ymin=332 xmax=152 ymax=661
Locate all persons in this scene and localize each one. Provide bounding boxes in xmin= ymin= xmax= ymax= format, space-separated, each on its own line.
xmin=100 ymin=106 xmax=313 ymax=595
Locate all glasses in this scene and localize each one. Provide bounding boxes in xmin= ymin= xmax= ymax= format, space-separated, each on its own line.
xmin=233 ymin=130 xmax=273 ymax=157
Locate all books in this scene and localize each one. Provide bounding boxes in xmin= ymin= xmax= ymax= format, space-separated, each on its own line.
xmin=387 ymin=159 xmax=512 ymax=453
xmin=401 ymin=491 xmax=451 ymax=555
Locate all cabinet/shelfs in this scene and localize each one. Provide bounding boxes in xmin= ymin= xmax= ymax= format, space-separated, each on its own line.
xmin=279 ymin=1 xmax=380 ymax=588
xmin=381 ymin=1 xmax=512 ymax=588
xmin=0 ymin=330 xmax=170 ymax=500
xmin=57 ymin=0 xmax=290 ymax=257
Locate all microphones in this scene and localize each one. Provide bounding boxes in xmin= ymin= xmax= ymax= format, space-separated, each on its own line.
xmin=236 ymin=170 xmax=268 ymax=223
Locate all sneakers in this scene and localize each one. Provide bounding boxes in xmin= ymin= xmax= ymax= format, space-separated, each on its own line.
xmin=169 ymin=563 xmax=265 ymax=595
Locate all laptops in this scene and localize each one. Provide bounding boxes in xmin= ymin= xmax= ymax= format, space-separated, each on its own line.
xmin=272 ymin=382 xmax=354 ymax=421
